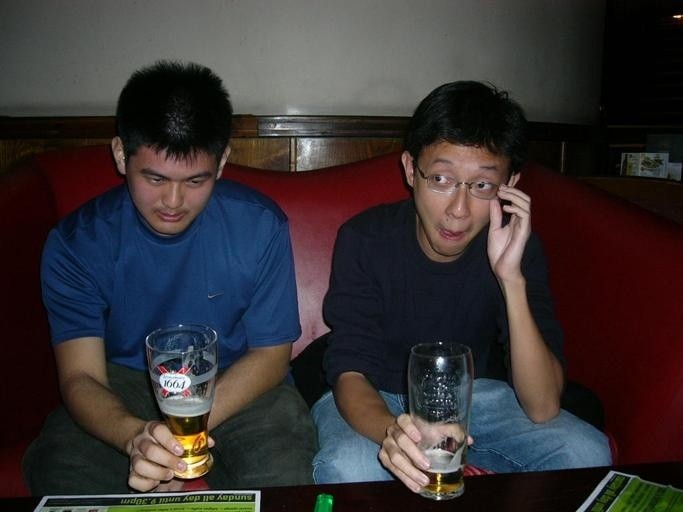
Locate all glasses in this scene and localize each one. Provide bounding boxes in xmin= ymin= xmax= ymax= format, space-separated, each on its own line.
xmin=413 ymin=157 xmax=513 ymax=200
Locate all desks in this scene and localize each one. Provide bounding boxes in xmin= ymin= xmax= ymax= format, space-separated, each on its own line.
xmin=0 ymin=458 xmax=683 ymax=512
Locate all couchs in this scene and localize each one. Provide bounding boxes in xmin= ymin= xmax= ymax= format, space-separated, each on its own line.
xmin=0 ymin=143 xmax=683 ymax=499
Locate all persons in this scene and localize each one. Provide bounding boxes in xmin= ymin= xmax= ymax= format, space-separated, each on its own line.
xmin=307 ymin=77 xmax=615 ymax=490
xmin=17 ymin=55 xmax=322 ymax=498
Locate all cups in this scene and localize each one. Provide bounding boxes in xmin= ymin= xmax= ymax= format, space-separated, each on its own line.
xmin=145 ymin=322 xmax=219 ymax=480
xmin=407 ymin=341 xmax=475 ymax=501
